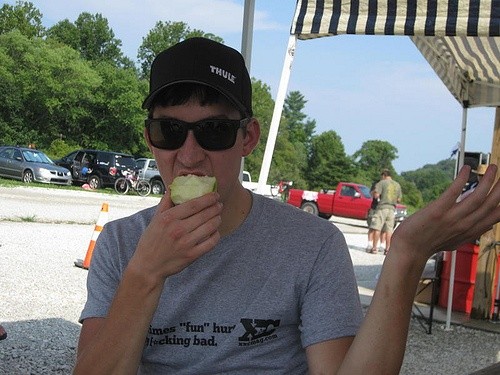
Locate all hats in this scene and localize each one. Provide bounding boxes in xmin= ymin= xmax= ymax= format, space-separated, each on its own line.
xmin=142 ymin=37 xmax=252 ymax=118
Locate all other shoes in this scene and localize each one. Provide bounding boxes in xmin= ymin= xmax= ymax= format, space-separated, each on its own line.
xmin=367 ymin=246 xmax=387 ymax=255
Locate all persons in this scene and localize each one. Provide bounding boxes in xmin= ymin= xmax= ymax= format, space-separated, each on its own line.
xmin=71 ymin=37 xmax=500 ymax=375
xmin=368 ymin=170 xmax=402 ymax=255
xmin=28 ymin=143 xmax=35 ymax=149
xmin=365 ymin=169 xmax=388 ymax=253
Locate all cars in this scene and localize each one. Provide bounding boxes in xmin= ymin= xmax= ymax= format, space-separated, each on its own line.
xmin=0 ymin=145 xmax=73 ymax=186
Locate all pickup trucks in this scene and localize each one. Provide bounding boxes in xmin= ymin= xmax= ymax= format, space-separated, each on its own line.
xmin=287 ymin=181 xmax=408 ymax=229
xmin=242 ymin=171 xmax=281 ymax=197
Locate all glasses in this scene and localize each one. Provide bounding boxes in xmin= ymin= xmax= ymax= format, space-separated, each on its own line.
xmin=144 ymin=118 xmax=250 ymax=151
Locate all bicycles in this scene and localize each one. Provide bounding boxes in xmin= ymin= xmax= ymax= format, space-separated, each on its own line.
xmin=113 ymin=172 xmax=151 ymax=197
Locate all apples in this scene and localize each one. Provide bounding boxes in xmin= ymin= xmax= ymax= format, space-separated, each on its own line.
xmin=170 ymin=174 xmax=216 ymax=205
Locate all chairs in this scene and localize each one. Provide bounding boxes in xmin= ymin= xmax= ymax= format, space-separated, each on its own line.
xmin=411 ymin=253 xmax=443 ymax=336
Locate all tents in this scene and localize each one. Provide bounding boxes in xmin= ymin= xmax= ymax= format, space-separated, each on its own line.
xmin=255 ymin=0 xmax=500 ymax=330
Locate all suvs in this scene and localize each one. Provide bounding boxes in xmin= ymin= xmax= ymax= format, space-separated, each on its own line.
xmin=53 ymin=148 xmax=137 ymax=190
xmin=134 ymin=157 xmax=166 ymax=195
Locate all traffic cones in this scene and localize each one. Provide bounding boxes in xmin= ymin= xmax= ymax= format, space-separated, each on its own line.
xmin=74 ymin=203 xmax=110 ymax=270
